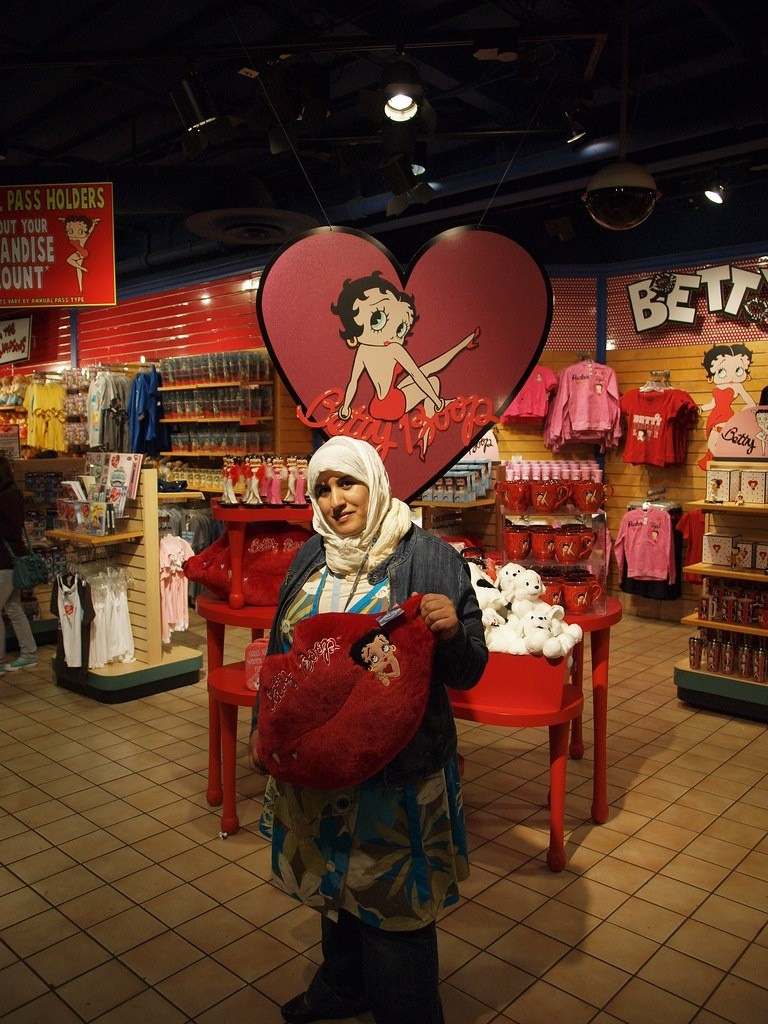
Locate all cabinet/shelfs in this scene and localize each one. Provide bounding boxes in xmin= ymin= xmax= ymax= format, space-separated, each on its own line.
xmin=159 ymin=346 xmax=282 ymax=457
xmin=673 ymin=461 xmax=768 ymax=720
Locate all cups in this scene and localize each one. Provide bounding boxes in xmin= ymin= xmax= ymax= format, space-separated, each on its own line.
xmin=529 ymin=567 xmax=602 ymax=615
xmin=697 ymin=585 xmax=768 ymax=630
xmin=504 ymin=524 xmax=598 ymax=564
xmin=688 ymin=627 xmax=768 ymax=683
xmin=506 ymin=457 xmax=602 ymax=483
xmin=495 ymin=480 xmax=613 ymax=514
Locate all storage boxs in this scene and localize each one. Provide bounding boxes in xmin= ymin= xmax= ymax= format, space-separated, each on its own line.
xmin=422 ymin=459 xmax=492 ymax=501
xmin=702 ymin=468 xmax=768 ymax=571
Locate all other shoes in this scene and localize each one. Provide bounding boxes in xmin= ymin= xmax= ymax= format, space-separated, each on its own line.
xmin=281 ymin=992 xmax=353 ymax=1024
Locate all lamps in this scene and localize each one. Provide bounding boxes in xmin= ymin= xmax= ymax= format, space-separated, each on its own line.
xmin=383 ymin=81 xmax=421 ymax=123
xmin=705 ymin=184 xmax=726 ymax=206
xmin=563 ymin=112 xmax=586 ymax=145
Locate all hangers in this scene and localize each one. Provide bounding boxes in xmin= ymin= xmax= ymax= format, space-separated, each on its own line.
xmin=639 ymin=369 xmax=673 ymax=394
xmin=627 ymin=488 xmax=680 ymax=514
xmin=159 ymin=502 xmax=223 ymax=539
xmin=64 ymin=545 xmax=135 ymax=593
xmin=426 ymin=512 xmax=473 ymax=538
xmin=30 ymin=361 xmax=160 ymax=386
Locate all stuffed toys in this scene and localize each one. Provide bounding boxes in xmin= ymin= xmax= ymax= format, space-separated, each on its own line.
xmin=467 ymin=561 xmax=584 ymax=658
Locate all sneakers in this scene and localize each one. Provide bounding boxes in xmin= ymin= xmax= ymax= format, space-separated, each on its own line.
xmin=0 ymin=667 xmax=6 ymax=675
xmin=5 ymin=657 xmax=38 ymax=671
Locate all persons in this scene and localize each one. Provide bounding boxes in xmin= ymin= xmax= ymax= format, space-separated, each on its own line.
xmin=247 ymin=434 xmax=488 ymax=1024
xmin=0 ymin=451 xmax=38 ymax=678
xmin=221 ymin=453 xmax=313 ymax=505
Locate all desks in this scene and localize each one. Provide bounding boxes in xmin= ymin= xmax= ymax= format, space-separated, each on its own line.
xmin=447 ymin=649 xmax=586 ymax=875
xmin=209 ymin=497 xmax=314 ymax=608
xmin=204 ymin=661 xmax=258 ymax=834
xmin=193 ymin=590 xmax=623 ymax=826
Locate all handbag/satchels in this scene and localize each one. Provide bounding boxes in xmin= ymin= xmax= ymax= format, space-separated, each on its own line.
xmin=13 ymin=554 xmax=48 ymax=590
